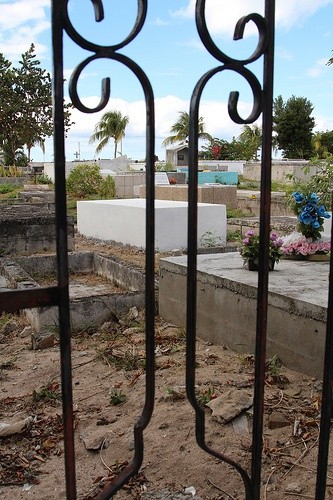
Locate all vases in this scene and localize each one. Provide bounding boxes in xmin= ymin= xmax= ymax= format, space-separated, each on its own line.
xmin=247 ymin=257 xmax=276 ymax=271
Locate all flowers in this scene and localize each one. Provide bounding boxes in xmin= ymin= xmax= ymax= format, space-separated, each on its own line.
xmin=293 ymin=191 xmax=331 ymax=229
xmin=284 ymin=238 xmax=331 ymax=256
xmin=237 ymin=230 xmax=289 ymax=258
